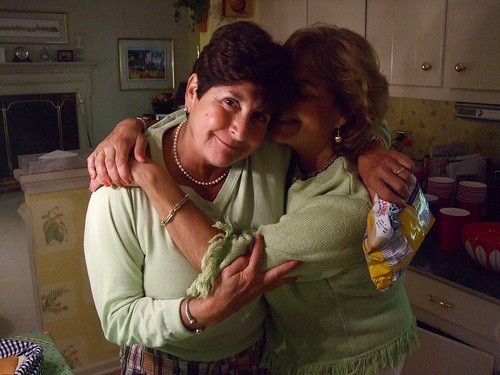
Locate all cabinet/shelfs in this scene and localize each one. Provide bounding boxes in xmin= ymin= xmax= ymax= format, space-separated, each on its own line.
xmin=398 ymin=269 xmax=500 ymax=375
xmin=207 ymin=0 xmax=500 ymax=104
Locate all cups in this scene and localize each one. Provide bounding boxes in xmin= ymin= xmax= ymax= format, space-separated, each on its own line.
xmin=426 ymin=176 xmax=456 ymax=215
xmin=423 ymin=194 xmax=440 ymax=216
xmin=439 ymin=207 xmax=470 ymax=252
xmin=456 ymin=181 xmax=488 ymax=222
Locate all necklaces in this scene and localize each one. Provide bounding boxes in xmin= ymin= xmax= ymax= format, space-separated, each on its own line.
xmin=173 ymin=119 xmax=231 ymax=185
xmin=292 ymin=155 xmax=337 ymax=181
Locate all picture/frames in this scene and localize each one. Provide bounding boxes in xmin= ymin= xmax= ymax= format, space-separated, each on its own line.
xmin=117 ymin=37 xmax=176 ymax=91
xmin=0 ymin=9 xmax=68 ymax=44
xmin=56 ymin=50 xmax=73 ymax=62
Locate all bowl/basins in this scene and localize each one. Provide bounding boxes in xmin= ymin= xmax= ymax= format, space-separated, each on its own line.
xmin=462 ymin=222 xmax=500 ymax=273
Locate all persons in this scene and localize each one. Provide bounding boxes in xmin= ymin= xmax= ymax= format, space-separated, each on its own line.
xmin=82 ymin=21 xmax=416 ymax=375
xmin=87 ymin=23 xmax=421 ymax=375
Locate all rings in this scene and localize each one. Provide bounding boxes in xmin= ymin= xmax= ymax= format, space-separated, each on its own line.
xmin=96 ymin=153 xmax=106 ymax=156
xmin=395 ymin=167 xmax=405 ymax=175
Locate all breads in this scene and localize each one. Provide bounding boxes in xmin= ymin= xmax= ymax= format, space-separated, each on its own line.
xmin=0 ymin=356 xmax=19 ymax=375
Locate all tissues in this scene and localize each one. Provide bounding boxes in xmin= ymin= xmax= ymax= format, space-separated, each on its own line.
xmin=16 ymin=149 xmax=90 ymax=176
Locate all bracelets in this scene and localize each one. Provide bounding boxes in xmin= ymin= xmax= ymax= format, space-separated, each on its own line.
xmin=161 ymin=194 xmax=191 ymax=226
xmin=185 ymin=297 xmax=207 ymax=335
xmin=134 ymin=115 xmax=148 ymax=130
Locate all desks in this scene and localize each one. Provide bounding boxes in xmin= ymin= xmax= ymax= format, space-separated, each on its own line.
xmin=0 ymin=332 xmax=76 ymax=375
xmin=12 ymin=166 xmax=129 ymax=375
